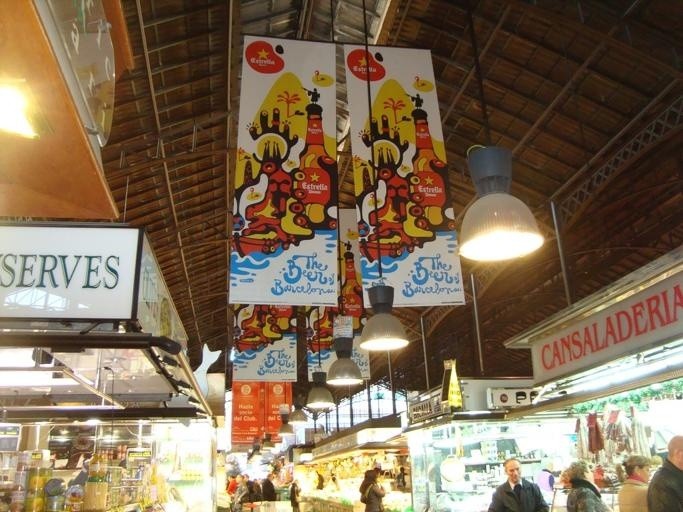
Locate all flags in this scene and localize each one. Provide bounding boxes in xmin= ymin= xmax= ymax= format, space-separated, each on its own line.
xmin=227 ymin=34 xmax=340 ymax=309
xmin=340 ymin=43 xmax=468 ymax=310
xmin=304 ymin=208 xmax=371 ymax=383
xmin=227 ymin=301 xmax=372 ymax=450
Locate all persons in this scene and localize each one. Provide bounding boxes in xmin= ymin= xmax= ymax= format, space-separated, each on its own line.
xmin=360 ymin=469 xmax=385 ymax=512
xmin=379 ymin=470 xmax=386 ymax=478
xmin=646 ymin=435 xmax=683 ymax=511
xmin=618 ymin=455 xmax=651 ymax=512
xmin=315 ymin=470 xmax=323 ymax=491
xmin=290 ymin=479 xmax=302 ymax=512
xmin=224 ymin=473 xmax=275 ymax=512
xmin=394 ymin=467 xmax=407 ymax=489
xmin=487 ymin=458 xmax=548 ymax=512
xmin=564 ymin=459 xmax=611 ymax=512
xmin=374 ymin=468 xmax=381 ymax=479
xmin=331 ymin=473 xmax=338 ymax=490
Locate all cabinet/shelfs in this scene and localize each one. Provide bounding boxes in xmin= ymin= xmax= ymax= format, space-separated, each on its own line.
xmin=1 ymin=426 xmax=153 ymax=511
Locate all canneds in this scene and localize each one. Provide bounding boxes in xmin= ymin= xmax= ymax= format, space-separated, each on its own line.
xmin=0 ymin=449 xmax=142 ymax=512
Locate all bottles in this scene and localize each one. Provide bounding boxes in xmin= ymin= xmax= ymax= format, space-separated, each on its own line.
xmin=82 ymin=464 xmax=108 ymax=511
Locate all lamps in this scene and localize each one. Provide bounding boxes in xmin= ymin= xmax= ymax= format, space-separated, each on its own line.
xmin=354 ymin=1 xmax=411 ymax=354
xmin=456 ymin=1 xmax=544 ymax=264
xmin=245 ymin=134 xmax=365 ymax=462
